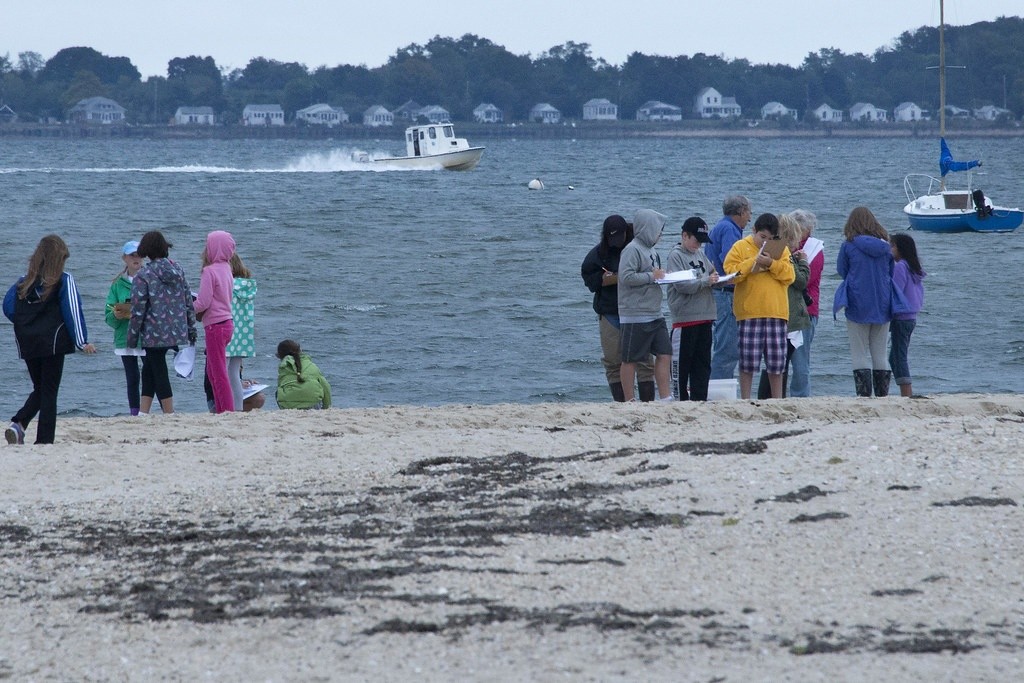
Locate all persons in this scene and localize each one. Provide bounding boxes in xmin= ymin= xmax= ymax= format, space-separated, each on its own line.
xmin=666 ymin=217 xmax=737 ymax=401
xmin=889 ymin=234 xmax=926 ymax=396
xmin=203 ymin=348 xmax=264 ymax=412
xmin=581 ymin=215 xmax=656 ymax=402
xmin=758 ymin=215 xmax=810 ymax=399
xmin=705 ymin=196 xmax=753 ymax=379
xmin=790 ymin=209 xmax=825 ymax=397
xmin=128 ymin=231 xmax=198 ymax=416
xmin=226 ymin=253 xmax=258 ymax=411
xmin=2 ymin=235 xmax=96 ymax=445
xmin=194 ymin=232 xmax=236 ymax=413
xmin=724 ymin=213 xmax=796 ymax=398
xmin=105 ymin=242 xmax=163 ymax=415
xmin=275 ymin=340 xmax=331 ymax=409
xmin=617 ymin=209 xmax=675 ymax=400
xmin=834 ymin=208 xmax=912 ymax=396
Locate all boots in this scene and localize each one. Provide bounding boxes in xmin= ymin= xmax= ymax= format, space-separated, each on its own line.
xmin=853 ymin=369 xmax=872 ymax=397
xmin=609 ymin=382 xmax=624 ymax=402
xmin=873 ymin=370 xmax=892 ymax=397
xmin=638 ymin=381 xmax=655 ymax=402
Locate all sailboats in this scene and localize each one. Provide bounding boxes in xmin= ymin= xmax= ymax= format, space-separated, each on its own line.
xmin=903 ymin=1 xmax=1024 ymax=233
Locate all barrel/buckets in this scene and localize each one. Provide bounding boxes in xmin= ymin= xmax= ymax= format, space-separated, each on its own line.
xmin=708 ymin=378 xmax=737 ymax=401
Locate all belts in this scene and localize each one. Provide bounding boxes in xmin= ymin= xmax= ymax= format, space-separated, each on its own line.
xmin=713 ymin=285 xmax=734 ymax=293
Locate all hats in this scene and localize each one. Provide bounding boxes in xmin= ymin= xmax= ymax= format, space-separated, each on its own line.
xmin=122 ymin=241 xmax=140 ymax=255
xmin=603 ymin=215 xmax=627 ymax=247
xmin=683 ymin=217 xmax=713 ymax=245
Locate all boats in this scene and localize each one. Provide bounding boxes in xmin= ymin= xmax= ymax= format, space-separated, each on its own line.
xmin=349 ymin=122 xmax=486 ymax=171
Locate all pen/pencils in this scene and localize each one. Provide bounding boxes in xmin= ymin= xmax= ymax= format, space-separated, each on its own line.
xmin=712 ymin=262 xmax=716 ymax=273
xmin=107 ymin=305 xmax=114 ymax=309
xmin=602 ymin=268 xmax=609 ymax=274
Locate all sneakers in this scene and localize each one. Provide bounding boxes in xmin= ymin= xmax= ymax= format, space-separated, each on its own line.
xmin=5 ymin=422 xmax=26 ymax=444
